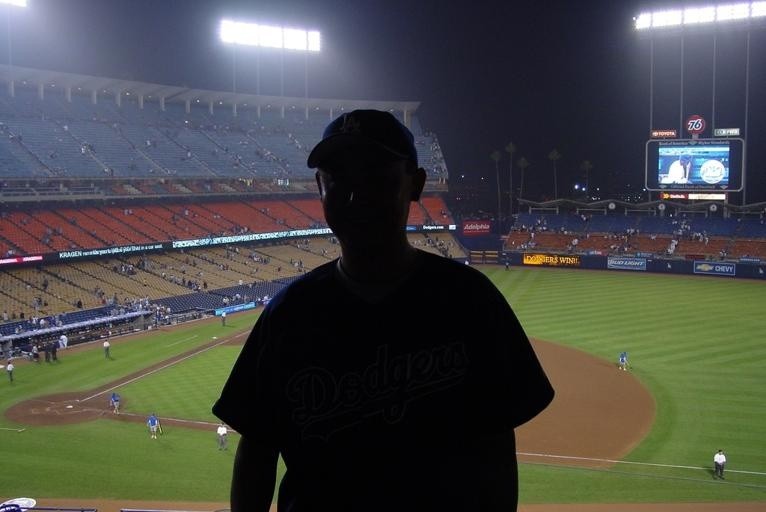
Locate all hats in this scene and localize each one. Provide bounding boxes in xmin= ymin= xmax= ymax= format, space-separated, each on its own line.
xmin=301 ymin=107 xmax=419 ymax=172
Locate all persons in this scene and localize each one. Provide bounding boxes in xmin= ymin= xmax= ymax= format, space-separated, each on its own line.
xmin=209 ymin=107 xmax=556 ymax=512
xmin=109 ymin=392 xmax=122 ymax=414
xmin=667 ymin=154 xmax=693 ymax=184
xmin=458 ymin=177 xmax=766 ymax=280
xmin=146 ymin=412 xmax=160 ymax=439
xmin=0 ymin=97 xmax=456 ymax=360
xmin=619 ymin=351 xmax=627 ymax=372
xmin=216 ymin=421 xmax=228 ymax=450
xmin=6 ymin=360 xmax=14 ymax=379
xmin=713 ymin=449 xmax=727 ymax=480
